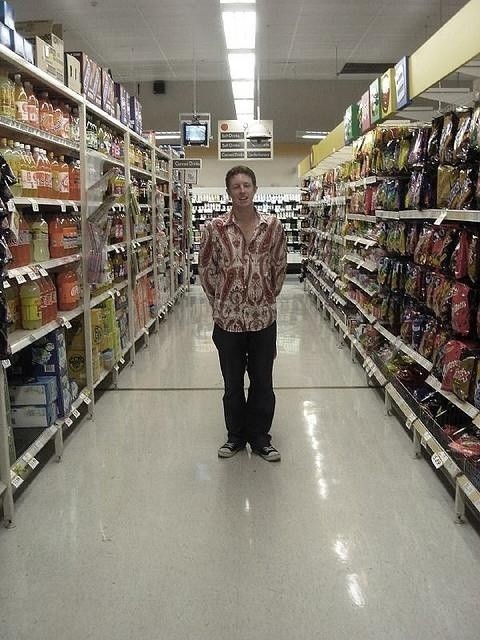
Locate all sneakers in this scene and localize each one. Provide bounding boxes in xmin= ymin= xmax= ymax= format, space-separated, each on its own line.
xmin=218 ymin=440 xmax=247 ymax=458
xmin=251 ymin=444 xmax=281 ymax=461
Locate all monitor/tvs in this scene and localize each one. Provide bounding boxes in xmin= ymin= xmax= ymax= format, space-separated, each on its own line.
xmin=184 ymin=124 xmax=207 ymax=145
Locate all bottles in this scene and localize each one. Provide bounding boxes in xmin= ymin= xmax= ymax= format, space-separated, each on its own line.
xmin=1 ymin=68 xmax=151 ymax=350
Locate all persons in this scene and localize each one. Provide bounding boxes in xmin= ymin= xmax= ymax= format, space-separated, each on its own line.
xmin=198 ymin=165 xmax=289 ymax=462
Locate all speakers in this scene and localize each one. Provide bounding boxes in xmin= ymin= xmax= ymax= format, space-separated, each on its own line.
xmin=153 ymin=81 xmax=164 ymax=94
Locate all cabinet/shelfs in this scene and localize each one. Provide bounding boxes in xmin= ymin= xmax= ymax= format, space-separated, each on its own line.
xmin=298 ymin=0 xmax=479 ymax=527
xmin=189 ymin=186 xmax=302 ymax=265
xmin=2 ymin=45 xmax=192 ymax=527
